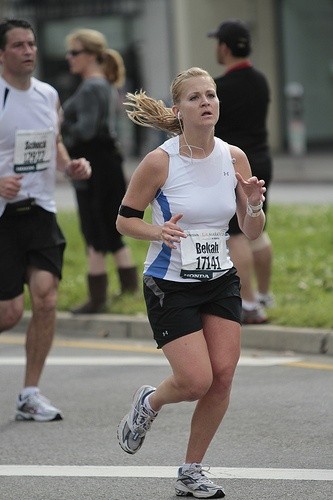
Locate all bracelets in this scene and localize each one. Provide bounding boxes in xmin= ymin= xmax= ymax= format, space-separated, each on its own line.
xmin=246 ymin=200 xmax=263 ymax=218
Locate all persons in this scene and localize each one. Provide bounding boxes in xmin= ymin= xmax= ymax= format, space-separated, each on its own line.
xmin=118 ymin=69 xmax=268 ymax=499
xmin=59 ymin=30 xmax=139 ymax=318
xmin=201 ymin=19 xmax=270 ymax=325
xmin=0 ymin=19 xmax=92 ymax=421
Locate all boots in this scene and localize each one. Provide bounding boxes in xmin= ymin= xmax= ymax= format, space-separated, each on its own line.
xmin=71 ymin=272 xmax=108 ymax=315
xmin=112 ymin=266 xmax=138 ymax=300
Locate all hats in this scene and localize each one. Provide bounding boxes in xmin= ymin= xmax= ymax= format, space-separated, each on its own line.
xmin=207 ymin=21 xmax=250 ymax=44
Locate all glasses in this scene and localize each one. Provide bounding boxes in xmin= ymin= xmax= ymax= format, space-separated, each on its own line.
xmin=66 ymin=47 xmax=90 ymax=56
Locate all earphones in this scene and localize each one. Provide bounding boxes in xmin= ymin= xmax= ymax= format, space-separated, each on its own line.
xmin=178 ymin=111 xmax=181 ymax=119
xmin=232 ymin=157 xmax=236 ymax=163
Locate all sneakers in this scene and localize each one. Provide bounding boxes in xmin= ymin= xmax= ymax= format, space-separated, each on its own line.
xmin=117 ymin=385 xmax=159 ymax=455
xmin=175 ymin=463 xmax=226 ymax=499
xmin=242 ymin=305 xmax=268 ymax=323
xmin=257 ymin=296 xmax=272 ymax=308
xmin=15 ymin=392 xmax=64 ymax=422
